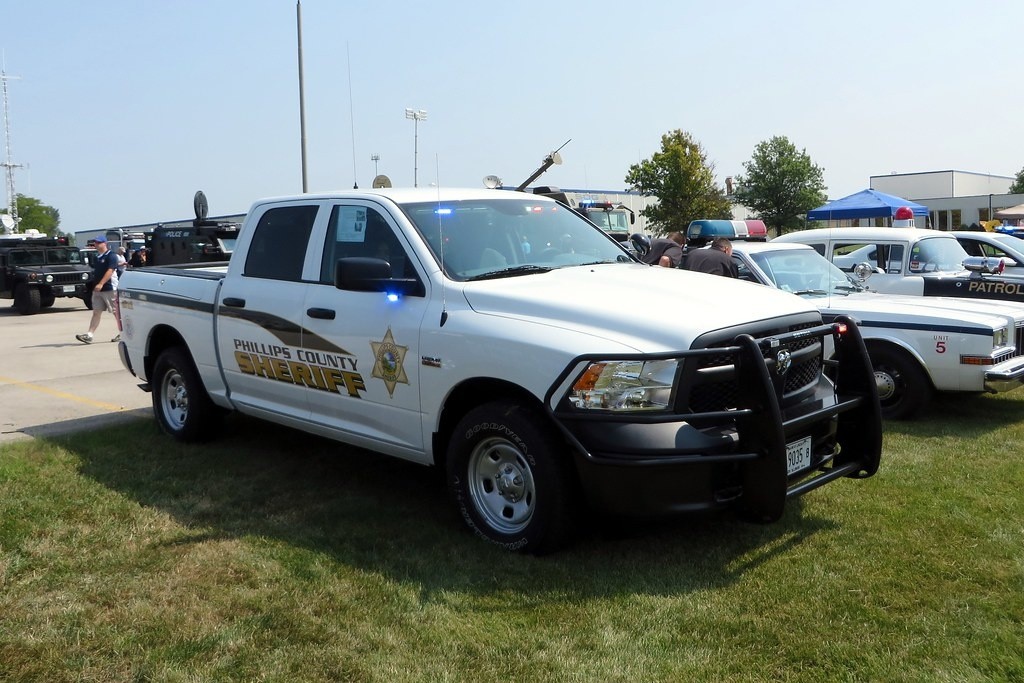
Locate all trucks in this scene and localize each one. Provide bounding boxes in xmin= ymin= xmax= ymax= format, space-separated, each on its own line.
xmin=573 ymin=200 xmax=636 ymax=250
xmin=81 ymin=227 xmax=152 ymax=263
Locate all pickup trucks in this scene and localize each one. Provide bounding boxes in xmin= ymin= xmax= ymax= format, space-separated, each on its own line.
xmin=110 ymin=185 xmax=887 ymax=555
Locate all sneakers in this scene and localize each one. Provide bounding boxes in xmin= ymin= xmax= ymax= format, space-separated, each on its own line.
xmin=111 ymin=335 xmax=120 ymax=342
xmin=76 ymin=333 xmax=93 ymax=344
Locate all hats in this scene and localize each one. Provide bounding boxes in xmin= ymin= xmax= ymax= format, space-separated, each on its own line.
xmin=140 ymin=246 xmax=145 ymax=250
xmin=93 ymin=236 xmax=107 ymax=242
xmin=117 ymin=247 xmax=126 ymax=253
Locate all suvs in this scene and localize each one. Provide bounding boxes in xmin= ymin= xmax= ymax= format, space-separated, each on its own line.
xmin=144 ymin=191 xmax=244 ymax=266
xmin=1 ymin=236 xmax=95 ymax=313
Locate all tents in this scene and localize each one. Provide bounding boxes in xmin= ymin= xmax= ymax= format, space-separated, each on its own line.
xmin=806 ymin=188 xmax=932 ymax=228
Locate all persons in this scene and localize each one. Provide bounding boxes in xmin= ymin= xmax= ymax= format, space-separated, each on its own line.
xmin=680 ymin=238 xmax=738 ymax=278
xmin=643 ymin=232 xmax=684 ymax=268
xmin=76 ymin=235 xmax=122 ymax=343
xmin=115 ymin=247 xmax=127 ymax=270
xmin=127 ymin=246 xmax=146 ymax=269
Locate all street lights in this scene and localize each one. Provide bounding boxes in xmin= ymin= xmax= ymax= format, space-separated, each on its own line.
xmin=370 ymin=153 xmax=381 ymax=178
xmin=405 ymin=107 xmax=429 ymax=187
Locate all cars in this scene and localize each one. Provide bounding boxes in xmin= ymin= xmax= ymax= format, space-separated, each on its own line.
xmin=682 ymin=219 xmax=1024 ymax=421
xmin=763 ymin=227 xmax=1024 ymax=332
xmin=71 ymin=249 xmax=90 ymax=265
xmin=836 ymin=230 xmax=1024 ymax=276
